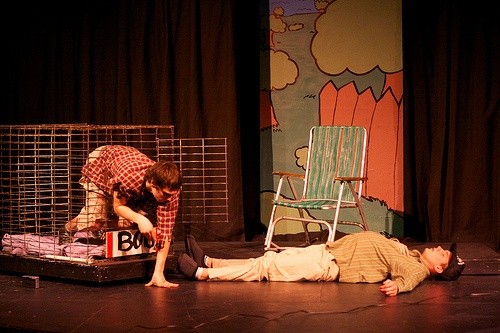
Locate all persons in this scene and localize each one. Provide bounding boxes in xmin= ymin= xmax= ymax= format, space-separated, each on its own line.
xmin=63 ymin=145 xmax=182 ymax=287
xmin=177 ymin=231 xmax=464 ymax=296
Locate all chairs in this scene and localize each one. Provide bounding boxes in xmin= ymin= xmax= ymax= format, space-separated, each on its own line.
xmin=264 ymin=125 xmax=368 ymax=251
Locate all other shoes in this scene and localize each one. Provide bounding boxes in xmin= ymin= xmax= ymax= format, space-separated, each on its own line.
xmin=187 ymin=234 xmax=209 ymax=268
xmin=58 ymin=229 xmax=72 ymax=246
xmin=177 ymin=253 xmax=199 ymax=281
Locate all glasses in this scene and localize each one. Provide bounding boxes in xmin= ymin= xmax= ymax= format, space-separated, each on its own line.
xmin=159 ymin=187 xmax=171 ymax=198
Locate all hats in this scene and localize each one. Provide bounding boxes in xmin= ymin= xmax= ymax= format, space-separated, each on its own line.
xmin=438 ymin=244 xmax=465 ymax=280
